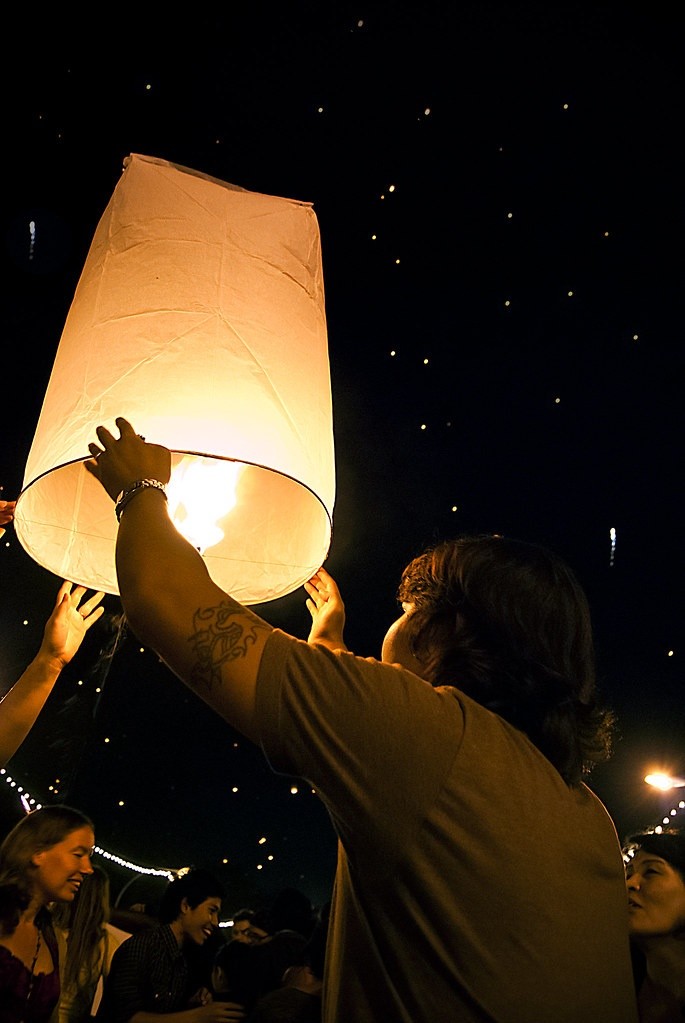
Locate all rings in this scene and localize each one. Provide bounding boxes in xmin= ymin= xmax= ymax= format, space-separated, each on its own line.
xmin=94 ymin=451 xmax=102 ymax=459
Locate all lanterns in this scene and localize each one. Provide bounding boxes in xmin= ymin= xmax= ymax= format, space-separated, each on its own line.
xmin=9 ymin=154 xmax=335 ymax=609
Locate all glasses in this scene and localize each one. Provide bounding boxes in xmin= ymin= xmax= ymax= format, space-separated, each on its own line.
xmin=245 ymin=930 xmax=264 ymax=940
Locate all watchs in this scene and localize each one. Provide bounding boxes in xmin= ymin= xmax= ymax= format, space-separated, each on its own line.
xmin=115 ymin=479 xmax=167 ymax=523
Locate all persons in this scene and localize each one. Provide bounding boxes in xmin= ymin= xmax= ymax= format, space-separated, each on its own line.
xmin=82 ymin=416 xmax=636 ymax=1023
xmin=0 ymin=500 xmax=105 ymax=772
xmin=619 ymin=832 xmax=685 ymax=1023
xmin=0 ymin=805 xmax=334 ymax=1023
